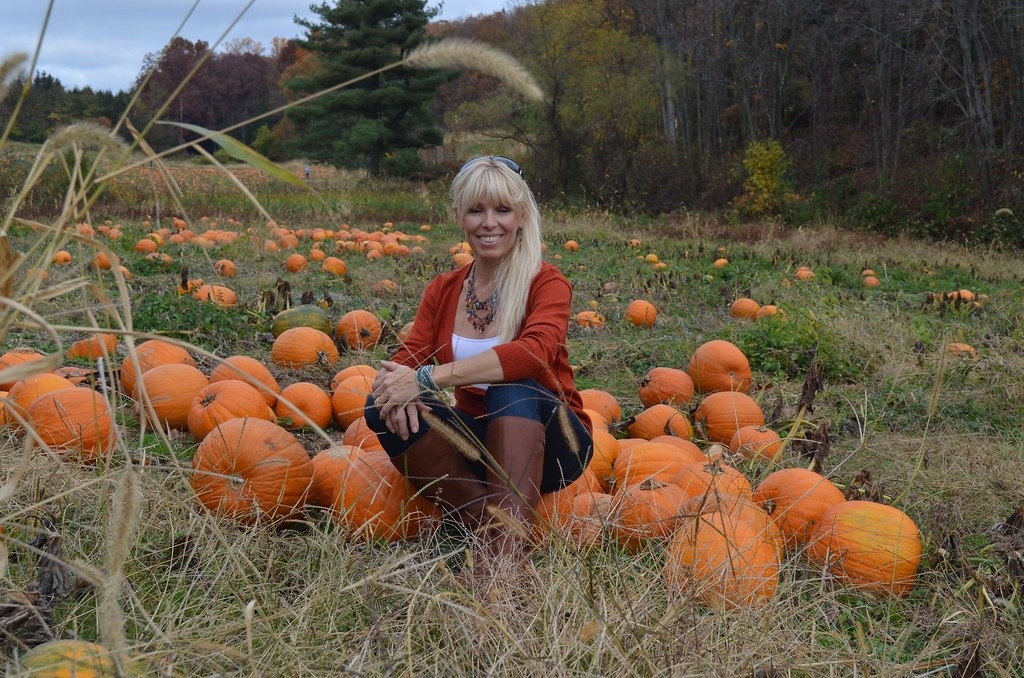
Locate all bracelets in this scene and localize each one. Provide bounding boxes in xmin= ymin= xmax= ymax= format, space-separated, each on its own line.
xmin=416 ymin=365 xmax=439 ymax=397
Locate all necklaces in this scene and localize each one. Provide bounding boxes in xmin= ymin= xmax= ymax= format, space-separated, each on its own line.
xmin=464 ymin=260 xmax=508 ymax=333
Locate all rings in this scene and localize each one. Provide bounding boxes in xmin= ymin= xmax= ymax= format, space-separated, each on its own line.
xmin=376 ymin=397 xmax=386 ymax=406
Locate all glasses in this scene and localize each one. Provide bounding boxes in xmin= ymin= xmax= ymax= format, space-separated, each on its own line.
xmin=462 ymin=155 xmax=524 ymax=181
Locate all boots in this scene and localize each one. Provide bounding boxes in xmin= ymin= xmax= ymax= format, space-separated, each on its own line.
xmin=390 ymin=428 xmax=495 ymax=591
xmin=488 ymin=416 xmax=545 ymax=603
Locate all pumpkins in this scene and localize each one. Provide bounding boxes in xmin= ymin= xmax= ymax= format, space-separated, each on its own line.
xmin=1 ymin=639 xmax=158 ymax=678
xmin=0 ymin=218 xmax=978 ymax=614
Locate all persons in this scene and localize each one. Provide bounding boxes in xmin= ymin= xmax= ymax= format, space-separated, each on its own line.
xmin=363 ymin=155 xmax=594 ymax=568
